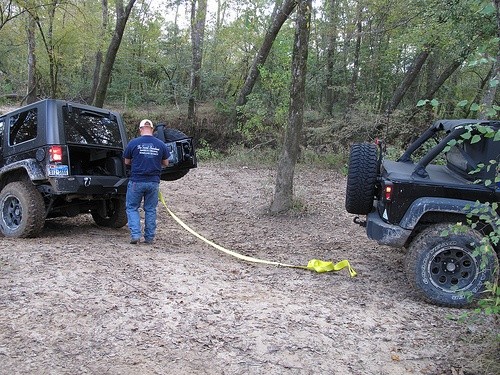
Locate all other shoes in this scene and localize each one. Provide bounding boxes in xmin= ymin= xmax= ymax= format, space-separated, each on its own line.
xmin=129 ymin=238 xmax=156 ymax=244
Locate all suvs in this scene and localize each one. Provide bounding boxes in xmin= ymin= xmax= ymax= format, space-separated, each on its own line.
xmin=345 ymin=119 xmax=499 ymax=308
xmin=0 ymin=99 xmax=196 ymax=237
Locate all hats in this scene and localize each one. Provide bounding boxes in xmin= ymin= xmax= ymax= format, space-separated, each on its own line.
xmin=139 ymin=119 xmax=153 ymax=128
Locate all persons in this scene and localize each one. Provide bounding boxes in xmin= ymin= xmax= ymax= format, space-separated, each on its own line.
xmin=123 ymin=119 xmax=169 ymax=245
